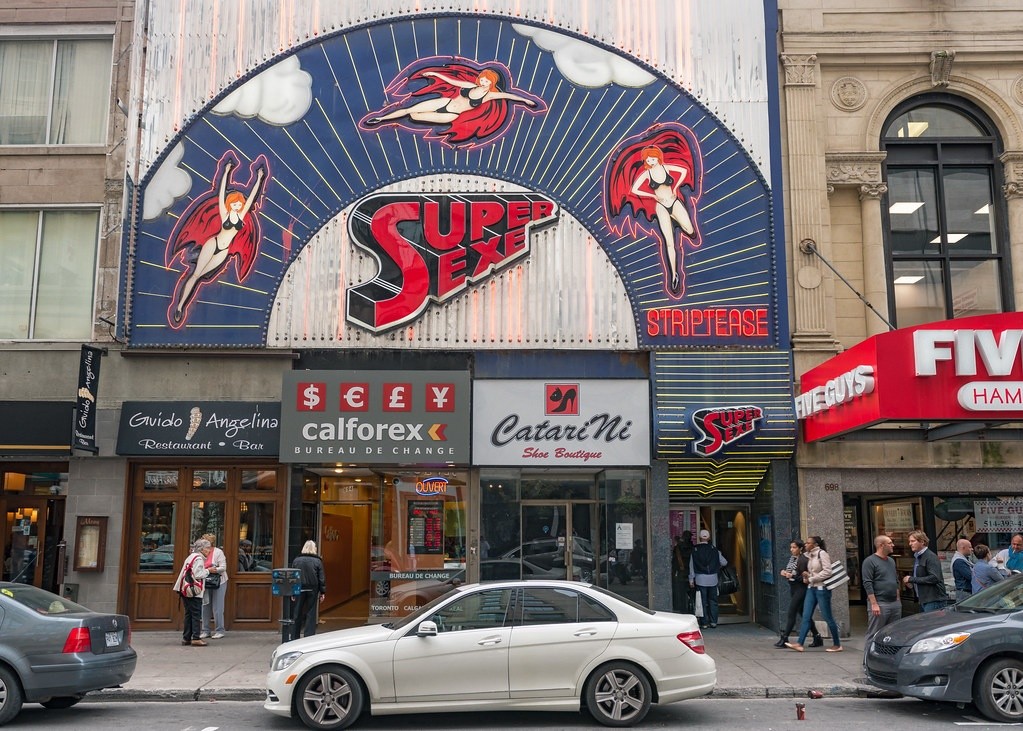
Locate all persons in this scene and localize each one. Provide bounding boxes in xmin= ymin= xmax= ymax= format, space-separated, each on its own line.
xmin=673 ymin=530 xmax=696 ymax=614
xmin=950 ymin=539 xmax=975 ymax=602
xmin=689 ymin=530 xmax=729 ymax=628
xmin=238 ymin=540 xmax=273 ymax=572
xmin=989 ymin=534 xmax=1023 ymax=574
xmin=971 ymin=544 xmax=1005 ymax=596
xmin=774 ymin=539 xmax=823 ymax=648
xmin=862 ymin=535 xmax=902 ymax=645
xmin=173 ymin=539 xmax=217 ymax=646
xmin=290 ymin=540 xmax=326 ymax=640
xmin=189 ymin=533 xmax=229 ymax=639
xmin=785 ymin=536 xmax=843 ymax=652
xmin=903 ymin=529 xmax=947 ymax=613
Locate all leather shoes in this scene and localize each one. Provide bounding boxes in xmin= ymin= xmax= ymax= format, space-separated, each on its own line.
xmin=181 ymin=638 xmax=192 ymax=646
xmin=191 ymin=639 xmax=208 ymax=647
xmin=774 ymin=634 xmax=788 ymax=646
xmin=808 ymin=639 xmax=823 ymax=647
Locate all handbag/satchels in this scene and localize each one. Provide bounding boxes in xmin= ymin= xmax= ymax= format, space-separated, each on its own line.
xmin=205 ymin=573 xmax=222 ymax=589
xmin=695 ymin=591 xmax=703 ymax=617
xmin=818 ymin=550 xmax=850 ymax=590
xmin=717 ymin=567 xmax=739 ymax=594
xmin=179 ymin=556 xmax=203 ymax=597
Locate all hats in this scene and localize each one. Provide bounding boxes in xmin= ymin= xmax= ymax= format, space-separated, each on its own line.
xmin=700 ymin=530 xmax=710 ymax=539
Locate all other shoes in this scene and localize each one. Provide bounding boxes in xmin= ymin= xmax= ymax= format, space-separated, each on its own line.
xmin=826 ymin=646 xmax=843 ymax=652
xmin=701 ymin=624 xmax=707 ymax=628
xmin=784 ymin=642 xmax=806 ymax=652
xmin=199 ymin=633 xmax=210 ymax=638
xmin=709 ymin=623 xmax=717 ymax=628
xmin=212 ymin=634 xmax=224 ymax=639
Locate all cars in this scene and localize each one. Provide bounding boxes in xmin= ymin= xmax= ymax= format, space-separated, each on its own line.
xmin=140 ymin=531 xmax=174 ymax=572
xmin=862 ymin=571 xmax=1023 ymax=725
xmin=368 ymin=530 xmax=613 ymax=618
xmin=0 ymin=578 xmax=139 ymax=729
xmin=262 ymin=578 xmax=719 ymax=731
